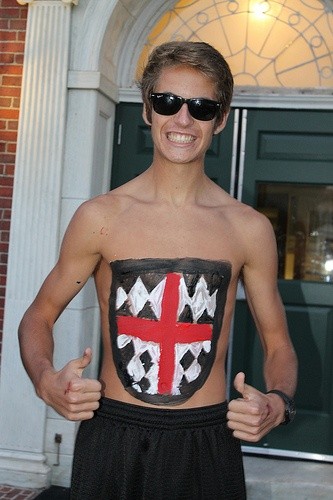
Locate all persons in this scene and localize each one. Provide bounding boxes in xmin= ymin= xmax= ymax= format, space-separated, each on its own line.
xmin=19 ymin=42 xmax=298 ymax=500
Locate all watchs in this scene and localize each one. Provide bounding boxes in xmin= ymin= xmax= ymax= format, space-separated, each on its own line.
xmin=264 ymin=390 xmax=296 ymax=425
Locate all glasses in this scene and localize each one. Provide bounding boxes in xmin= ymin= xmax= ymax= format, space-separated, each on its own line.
xmin=147 ymin=91 xmax=221 ymax=123
